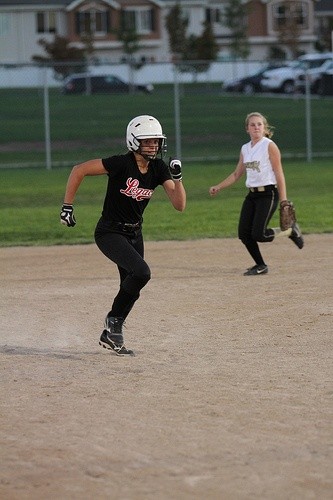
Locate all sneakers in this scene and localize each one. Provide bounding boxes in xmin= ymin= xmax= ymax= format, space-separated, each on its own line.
xmin=289 ymin=225 xmax=303 ymax=250
xmin=99 ymin=330 xmax=134 ymax=357
xmin=243 ymin=263 xmax=268 ymax=275
xmin=104 ymin=312 xmax=124 ymax=346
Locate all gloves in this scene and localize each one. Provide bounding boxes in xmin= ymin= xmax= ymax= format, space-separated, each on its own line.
xmin=60 ymin=203 xmax=76 ymax=227
xmin=169 ymin=157 xmax=182 ymax=181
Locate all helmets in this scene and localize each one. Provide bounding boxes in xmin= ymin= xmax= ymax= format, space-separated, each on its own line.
xmin=126 ymin=114 xmax=168 ymax=162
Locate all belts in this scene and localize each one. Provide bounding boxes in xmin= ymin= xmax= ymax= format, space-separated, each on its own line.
xmin=251 ymin=186 xmax=277 ymax=192
xmin=111 ymin=221 xmax=142 ymax=230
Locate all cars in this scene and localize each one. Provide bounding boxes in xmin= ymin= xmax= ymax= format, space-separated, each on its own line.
xmin=223 ymin=52 xmax=333 ymax=96
xmin=62 ymin=72 xmax=154 ymax=95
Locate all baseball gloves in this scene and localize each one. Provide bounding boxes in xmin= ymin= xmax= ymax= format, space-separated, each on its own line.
xmin=279 ymin=200 xmax=296 ymax=232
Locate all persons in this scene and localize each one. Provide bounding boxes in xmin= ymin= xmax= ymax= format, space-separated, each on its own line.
xmin=61 ymin=115 xmax=186 ymax=355
xmin=210 ymin=112 xmax=304 ymax=275
